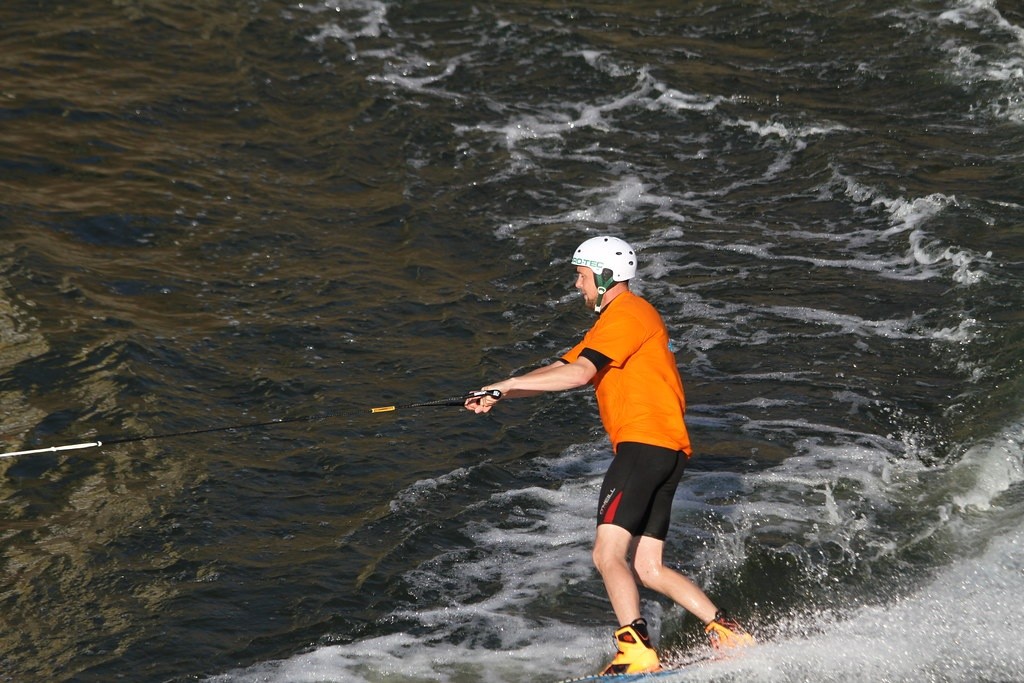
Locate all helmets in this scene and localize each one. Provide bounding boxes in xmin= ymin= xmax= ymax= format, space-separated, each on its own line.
xmin=571 ymin=235 xmax=637 ymax=281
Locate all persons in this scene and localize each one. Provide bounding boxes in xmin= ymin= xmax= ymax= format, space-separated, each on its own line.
xmin=465 ymin=236 xmax=752 ymax=672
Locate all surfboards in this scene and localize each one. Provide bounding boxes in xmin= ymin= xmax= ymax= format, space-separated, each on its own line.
xmin=553 ymin=653 xmax=719 ymax=683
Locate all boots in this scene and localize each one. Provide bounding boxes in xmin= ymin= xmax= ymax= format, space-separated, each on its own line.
xmin=601 ymin=618 xmax=660 ymax=677
xmin=705 ymin=608 xmax=756 ymax=657
